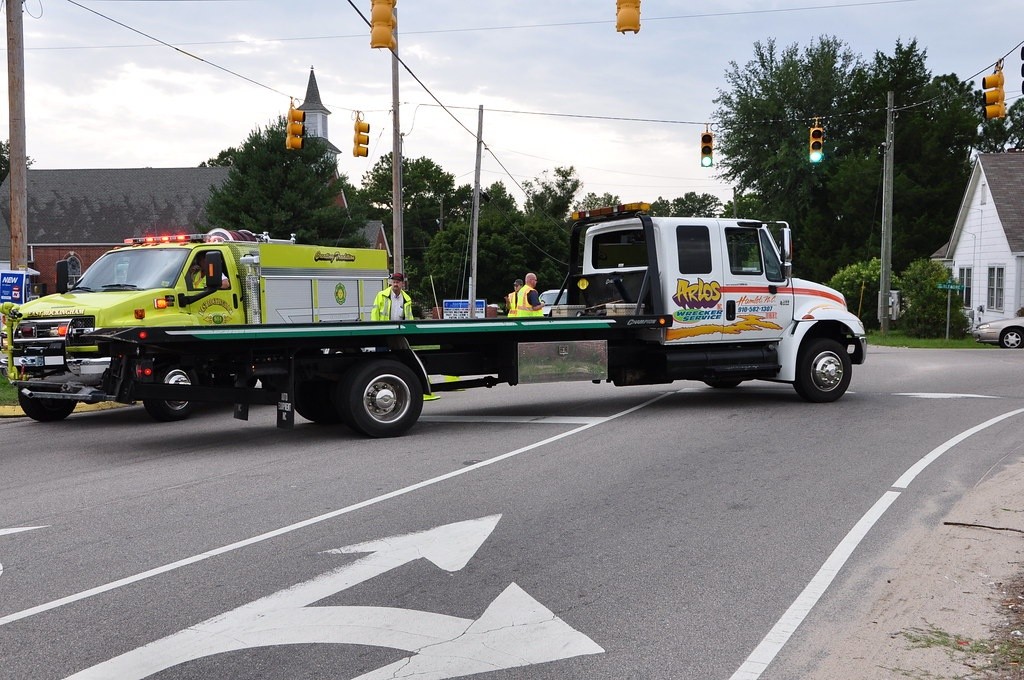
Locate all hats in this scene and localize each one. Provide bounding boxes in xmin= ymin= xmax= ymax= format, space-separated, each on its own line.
xmin=390 ymin=273 xmax=405 ymax=281
xmin=514 ymin=279 xmax=523 ymax=285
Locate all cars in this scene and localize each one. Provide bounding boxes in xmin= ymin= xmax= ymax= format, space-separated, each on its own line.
xmin=974 ymin=316 xmax=1024 ymax=349
xmin=538 ymin=289 xmax=567 ymax=316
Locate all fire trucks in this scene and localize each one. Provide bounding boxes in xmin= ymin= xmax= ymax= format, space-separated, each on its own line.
xmin=0 ymin=227 xmax=388 ymax=423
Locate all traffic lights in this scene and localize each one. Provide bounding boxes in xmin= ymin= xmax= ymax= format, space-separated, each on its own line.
xmin=1021 ymin=46 xmax=1024 ymax=95
xmin=982 ymin=71 xmax=1008 ymax=118
xmin=354 ymin=121 xmax=369 ymax=158
xmin=809 ymin=128 xmax=823 ymax=162
xmin=701 ymin=133 xmax=713 ymax=167
xmin=285 ymin=108 xmax=306 ymax=149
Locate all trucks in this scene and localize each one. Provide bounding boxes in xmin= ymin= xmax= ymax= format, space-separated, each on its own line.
xmin=171 ymin=203 xmax=870 ymax=440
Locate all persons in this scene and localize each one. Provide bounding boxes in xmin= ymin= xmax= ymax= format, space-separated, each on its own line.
xmin=504 ymin=279 xmax=524 ymax=317
xmin=517 ymin=273 xmax=545 ymax=317
xmin=189 ymin=253 xmax=230 ymax=289
xmin=371 ymin=272 xmax=414 ymax=321
